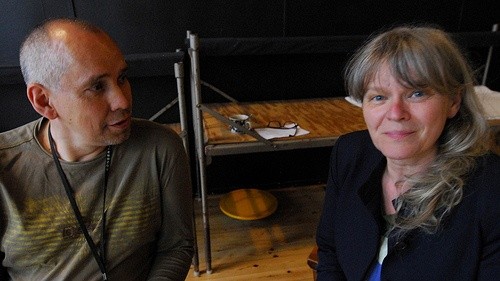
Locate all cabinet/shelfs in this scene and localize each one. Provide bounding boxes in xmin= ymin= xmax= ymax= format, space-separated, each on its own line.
xmin=187 ymin=23 xmax=500 ymax=275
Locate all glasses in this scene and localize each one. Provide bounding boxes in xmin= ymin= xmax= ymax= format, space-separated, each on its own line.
xmin=267 ymin=120 xmax=299 ymax=136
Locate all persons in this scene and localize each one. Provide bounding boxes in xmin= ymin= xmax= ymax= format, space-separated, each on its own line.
xmin=0 ymin=17 xmax=196 ymax=281
xmin=315 ymin=26 xmax=500 ymax=281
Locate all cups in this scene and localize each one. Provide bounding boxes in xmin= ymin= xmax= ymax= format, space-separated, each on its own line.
xmin=229 ymin=115 xmax=251 ymax=133
